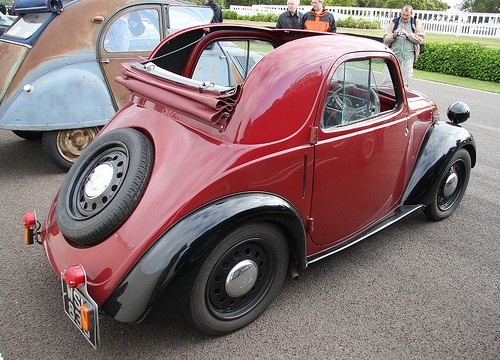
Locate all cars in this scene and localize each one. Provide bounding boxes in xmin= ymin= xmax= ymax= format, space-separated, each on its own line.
xmin=0 ymin=0 xmax=238 ymax=170
xmin=22 ymin=21 xmax=478 ymax=351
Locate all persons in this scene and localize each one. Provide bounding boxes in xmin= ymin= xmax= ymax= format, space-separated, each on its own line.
xmin=380 ymin=5 xmax=425 ymax=89
xmin=202 ymin=0 xmax=223 ymax=24
xmin=302 ymin=0 xmax=336 ymax=33
xmin=276 ymin=0 xmax=304 ymax=29
